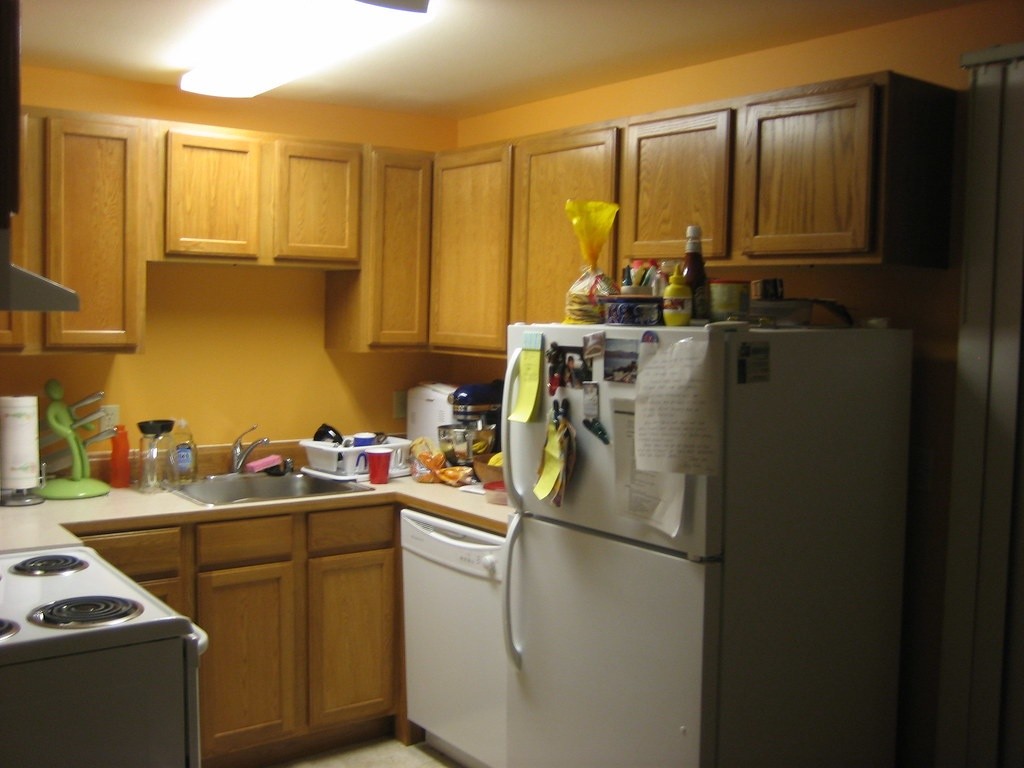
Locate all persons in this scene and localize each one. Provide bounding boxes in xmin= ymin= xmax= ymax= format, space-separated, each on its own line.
xmin=45 ymin=378 xmax=96 ymax=482
xmin=565 ymin=357 xmax=584 ymax=389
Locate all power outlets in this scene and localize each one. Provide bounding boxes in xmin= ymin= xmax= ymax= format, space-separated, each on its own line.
xmin=99 ymin=404 xmax=120 ymax=433
xmin=393 ymin=390 xmax=407 ymax=419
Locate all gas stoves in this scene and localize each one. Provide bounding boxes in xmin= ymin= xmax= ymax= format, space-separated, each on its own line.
xmin=0 ymin=546 xmax=194 ymax=666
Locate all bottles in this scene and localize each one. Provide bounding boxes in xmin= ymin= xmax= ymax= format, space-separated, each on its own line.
xmin=663 ymin=263 xmax=692 ymax=327
xmin=138 ymin=420 xmax=177 ymax=493
xmin=707 ymin=278 xmax=750 ymax=321
xmin=679 ymin=223 xmax=712 ymax=320
xmin=110 ymin=425 xmax=130 ymax=487
xmin=629 ymin=259 xmax=669 ymax=296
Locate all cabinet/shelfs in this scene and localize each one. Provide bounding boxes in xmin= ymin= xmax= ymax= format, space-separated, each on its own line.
xmin=0 ymin=68 xmax=971 ymax=361
xmin=77 ymin=502 xmax=425 ymax=768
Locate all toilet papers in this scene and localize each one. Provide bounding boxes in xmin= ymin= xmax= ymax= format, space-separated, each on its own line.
xmin=0 ymin=395 xmax=40 ymax=490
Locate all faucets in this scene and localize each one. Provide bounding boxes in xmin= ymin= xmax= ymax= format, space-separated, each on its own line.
xmin=230 ymin=423 xmax=270 ymax=474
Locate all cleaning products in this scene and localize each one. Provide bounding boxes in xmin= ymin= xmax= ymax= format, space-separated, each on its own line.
xmin=167 ymin=419 xmax=198 ymax=485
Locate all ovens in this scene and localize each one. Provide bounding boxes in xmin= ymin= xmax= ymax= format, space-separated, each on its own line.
xmin=0 ymin=622 xmax=210 ymax=768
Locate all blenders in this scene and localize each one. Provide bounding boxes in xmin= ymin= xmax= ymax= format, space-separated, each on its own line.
xmin=437 ymin=378 xmax=504 ymax=482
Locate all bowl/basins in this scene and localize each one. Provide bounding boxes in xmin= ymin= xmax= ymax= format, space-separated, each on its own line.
xmin=472 ymin=453 xmax=502 ymax=483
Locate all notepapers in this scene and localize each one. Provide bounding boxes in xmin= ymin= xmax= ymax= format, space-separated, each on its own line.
xmin=506 ymin=332 xmax=545 ymax=424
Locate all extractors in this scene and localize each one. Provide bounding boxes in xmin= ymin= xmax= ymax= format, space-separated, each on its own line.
xmin=0 ymin=0 xmax=82 ymax=312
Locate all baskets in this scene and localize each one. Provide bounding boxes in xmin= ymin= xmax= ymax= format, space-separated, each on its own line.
xmin=299 ymin=436 xmax=412 ymax=475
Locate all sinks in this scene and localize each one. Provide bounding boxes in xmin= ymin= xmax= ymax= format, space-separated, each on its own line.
xmin=173 ymin=468 xmax=374 ymax=507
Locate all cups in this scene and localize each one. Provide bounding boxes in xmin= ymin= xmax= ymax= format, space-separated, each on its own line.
xmin=365 ymin=447 xmax=394 ymax=484
xmin=620 ymin=286 xmax=653 ymax=295
xmin=353 ymin=432 xmax=377 ymax=467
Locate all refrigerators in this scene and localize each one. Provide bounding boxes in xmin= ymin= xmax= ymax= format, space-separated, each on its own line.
xmin=500 ymin=323 xmax=916 ymax=768
